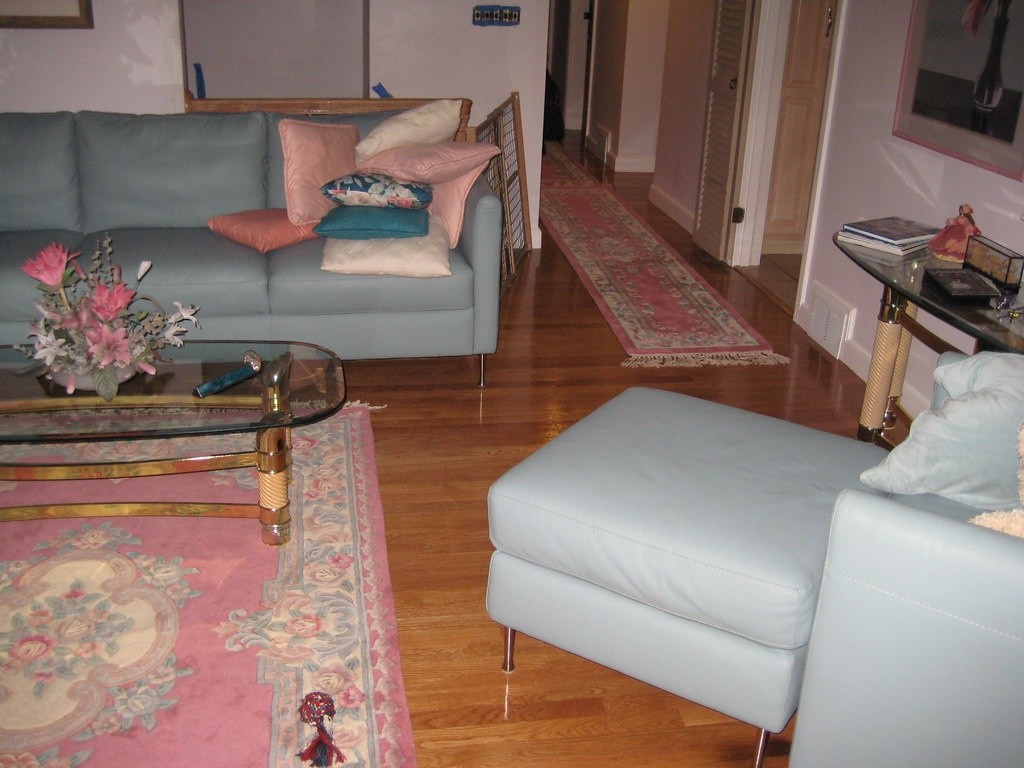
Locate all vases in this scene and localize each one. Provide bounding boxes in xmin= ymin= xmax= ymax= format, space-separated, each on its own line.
xmin=50 ymin=364 xmax=137 ymax=390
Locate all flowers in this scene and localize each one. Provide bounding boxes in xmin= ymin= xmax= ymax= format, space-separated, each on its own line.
xmin=13 ymin=229 xmax=203 ymax=403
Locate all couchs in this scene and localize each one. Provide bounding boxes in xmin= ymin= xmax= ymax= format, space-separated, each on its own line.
xmin=789 ymin=352 xmax=1024 ymax=768
xmin=0 ymin=111 xmax=503 ymax=386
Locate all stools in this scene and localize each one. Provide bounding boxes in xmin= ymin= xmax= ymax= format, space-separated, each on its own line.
xmin=484 ymin=386 xmax=891 ymax=768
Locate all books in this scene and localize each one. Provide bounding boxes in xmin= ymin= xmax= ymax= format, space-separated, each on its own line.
xmin=837 ymin=216 xmax=942 ymax=257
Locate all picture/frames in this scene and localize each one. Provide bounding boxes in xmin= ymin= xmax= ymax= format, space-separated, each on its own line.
xmin=892 ymin=0 xmax=1024 ymax=182
xmin=0 ymin=0 xmax=94 ymax=30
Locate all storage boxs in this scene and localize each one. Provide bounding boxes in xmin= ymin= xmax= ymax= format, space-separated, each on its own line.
xmin=923 ymin=269 xmax=1000 ymax=306
xmin=963 ymin=234 xmax=1024 ymax=296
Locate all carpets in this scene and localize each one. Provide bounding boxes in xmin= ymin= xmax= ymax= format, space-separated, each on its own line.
xmin=0 ymin=392 xmax=421 ymax=768
xmin=540 ymin=142 xmax=791 ymax=369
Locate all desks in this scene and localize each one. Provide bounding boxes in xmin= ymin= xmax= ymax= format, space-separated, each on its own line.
xmin=832 ymin=230 xmax=1024 ymax=443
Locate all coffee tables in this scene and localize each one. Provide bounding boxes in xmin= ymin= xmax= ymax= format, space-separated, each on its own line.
xmin=0 ymin=340 xmax=347 ymax=546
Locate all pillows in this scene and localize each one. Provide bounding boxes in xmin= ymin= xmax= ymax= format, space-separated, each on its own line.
xmin=318 ymin=174 xmax=435 ymax=210
xmin=354 ymin=99 xmax=462 ymax=167
xmin=859 ymin=351 xmax=1024 ymax=510
xmin=312 ymin=208 xmax=428 ymax=239
xmin=278 ymin=118 xmax=360 ymax=225
xmin=207 ymin=207 xmax=322 ymax=253
xmin=356 ymin=143 xmax=501 ymax=183
xmin=320 ymin=224 xmax=454 ymax=278
xmin=431 ymin=161 xmax=488 ymax=249
